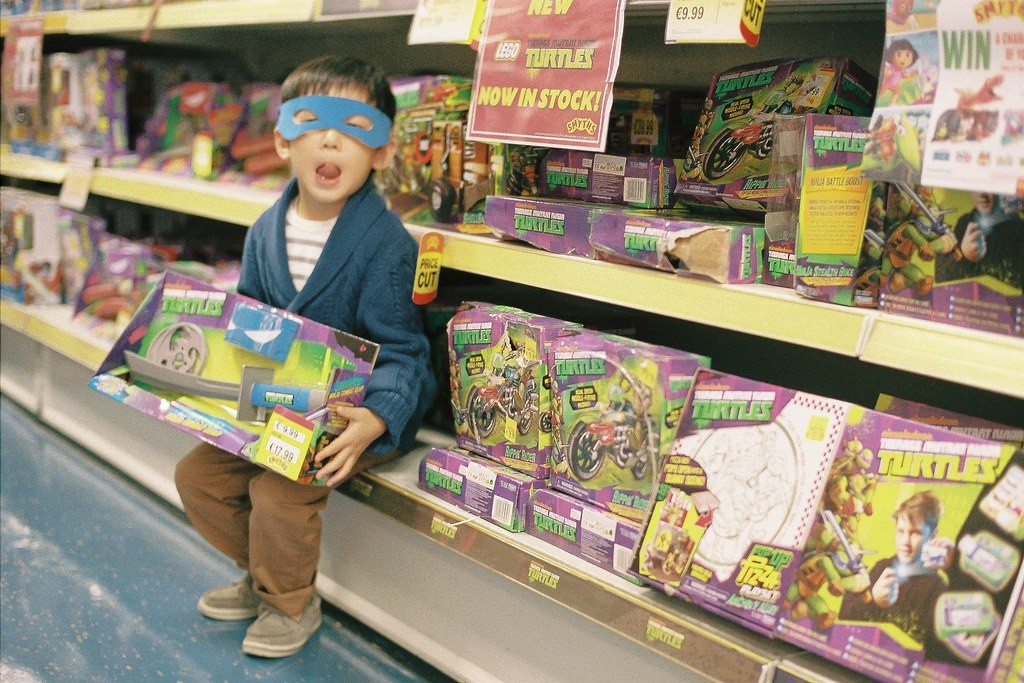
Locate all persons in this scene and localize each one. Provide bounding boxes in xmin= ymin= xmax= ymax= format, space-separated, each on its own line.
xmin=174 ymin=55 xmax=437 ymax=657
xmin=834 ymin=493 xmax=953 ymax=649
xmin=938 ymin=190 xmax=1024 ymax=290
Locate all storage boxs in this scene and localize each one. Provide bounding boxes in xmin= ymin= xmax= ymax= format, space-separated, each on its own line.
xmin=89 ymin=272 xmax=1024 ymax=683
xmin=0 ymin=44 xmax=1024 ymax=349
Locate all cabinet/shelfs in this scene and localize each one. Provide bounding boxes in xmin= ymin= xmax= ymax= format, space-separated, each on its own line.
xmin=0 ymin=0 xmax=1024 ymax=683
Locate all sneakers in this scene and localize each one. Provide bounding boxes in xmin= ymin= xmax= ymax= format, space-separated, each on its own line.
xmin=198 ymin=575 xmax=260 ymax=620
xmin=243 ymin=595 xmax=321 ymax=658
